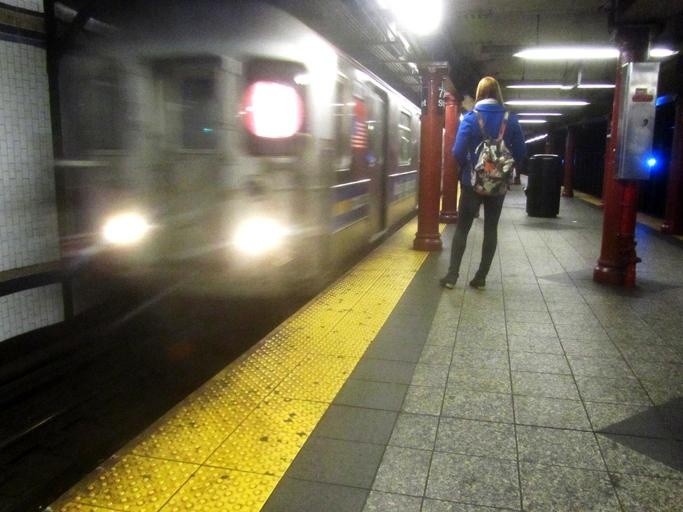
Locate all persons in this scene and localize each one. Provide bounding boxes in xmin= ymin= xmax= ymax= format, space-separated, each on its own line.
xmin=439 ymin=76 xmax=526 ymax=290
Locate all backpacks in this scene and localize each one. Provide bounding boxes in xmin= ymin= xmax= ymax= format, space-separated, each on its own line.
xmin=469 ymin=106 xmax=515 ymax=198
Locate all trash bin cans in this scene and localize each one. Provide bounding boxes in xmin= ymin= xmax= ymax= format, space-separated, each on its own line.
xmin=525 ymin=154 xmax=562 ymax=218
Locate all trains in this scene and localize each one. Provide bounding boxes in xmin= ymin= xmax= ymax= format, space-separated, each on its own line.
xmin=523 ymin=82 xmax=683 ymax=225
xmin=54 ymin=10 xmax=447 ymax=343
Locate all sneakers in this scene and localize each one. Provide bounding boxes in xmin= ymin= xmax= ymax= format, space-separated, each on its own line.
xmin=470 ymin=271 xmax=487 ymax=290
xmin=439 ymin=272 xmax=459 ymax=289
